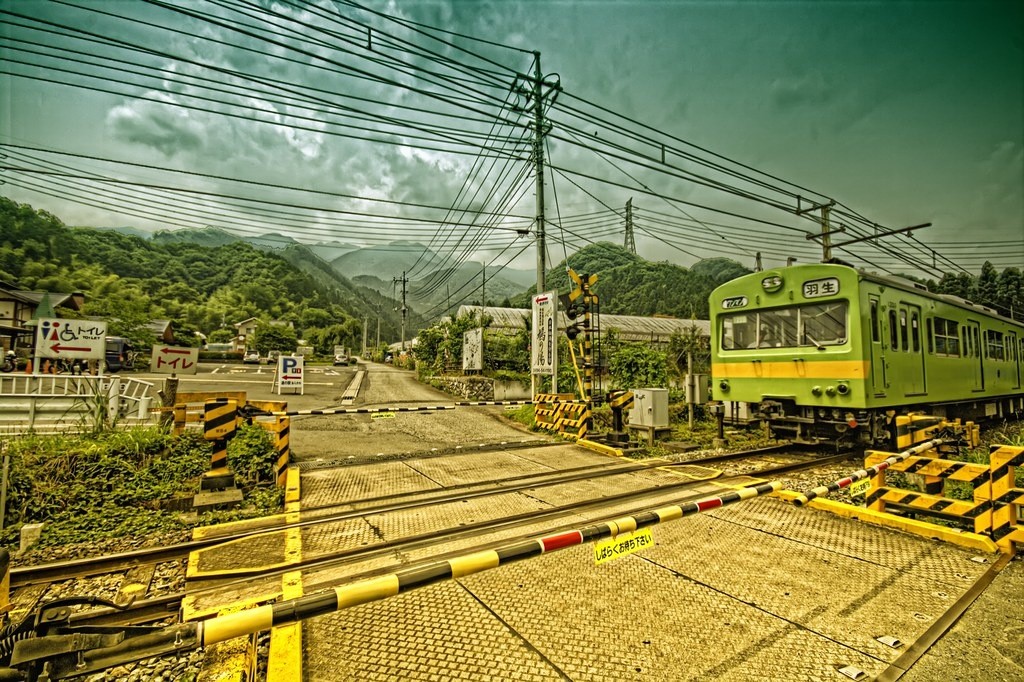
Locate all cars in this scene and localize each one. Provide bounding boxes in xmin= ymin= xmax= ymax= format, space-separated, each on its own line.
xmin=384 ymin=356 xmax=393 ymax=363
xmin=291 ymin=353 xmax=306 ymax=366
xmin=333 ymin=354 xmax=350 ymax=367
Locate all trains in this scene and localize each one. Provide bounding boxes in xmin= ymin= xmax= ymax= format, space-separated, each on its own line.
xmin=708 ymin=252 xmax=1024 ymax=446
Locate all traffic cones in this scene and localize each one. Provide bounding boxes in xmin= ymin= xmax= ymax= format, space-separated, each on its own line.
xmin=25 ymin=360 xmax=33 ymax=374
xmin=44 ymin=360 xmax=50 ymax=374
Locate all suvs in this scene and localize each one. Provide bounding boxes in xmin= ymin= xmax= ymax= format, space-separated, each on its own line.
xmin=267 ymin=351 xmax=281 ymax=363
xmin=243 ymin=350 xmax=260 ymax=363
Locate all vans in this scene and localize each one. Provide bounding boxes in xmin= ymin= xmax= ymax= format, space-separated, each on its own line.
xmin=50 ymin=337 xmax=134 ymax=373
